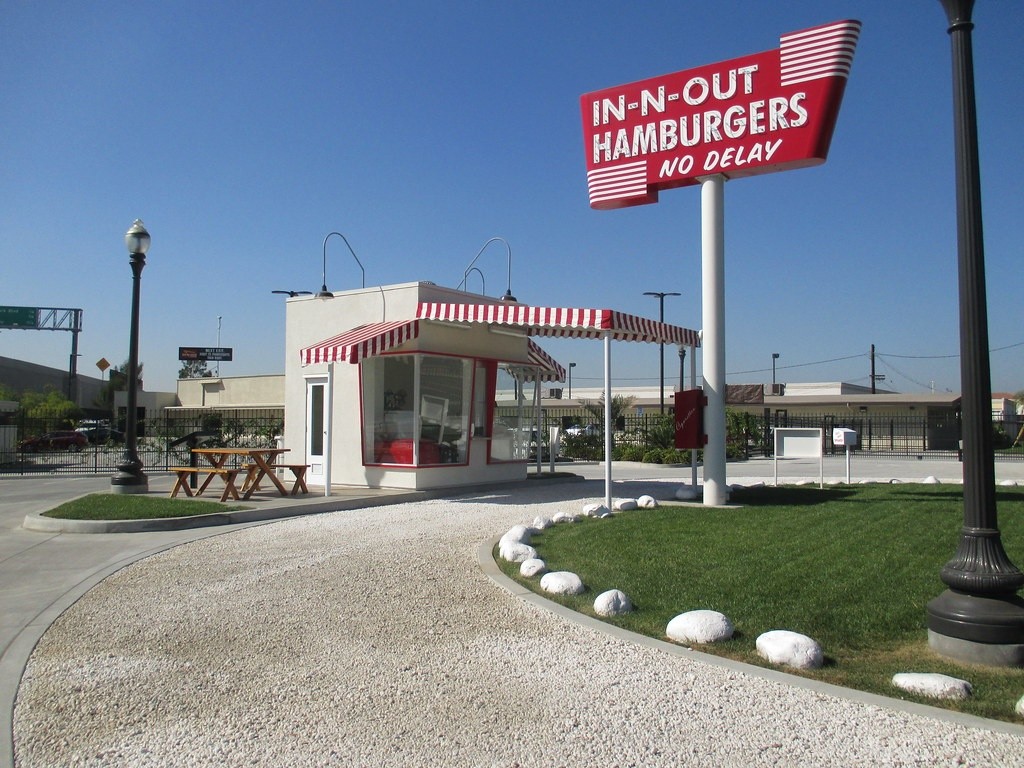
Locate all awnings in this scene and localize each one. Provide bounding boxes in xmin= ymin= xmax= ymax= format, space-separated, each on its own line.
xmin=505 ymin=337 xmax=565 ymax=475
xmin=300 ymin=320 xmax=419 ymax=497
xmin=420 ymin=302 xmax=700 ymax=512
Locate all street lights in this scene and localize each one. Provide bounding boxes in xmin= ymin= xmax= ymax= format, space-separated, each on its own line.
xmin=271 ymin=289 xmax=314 ymax=299
xmin=642 ymin=291 xmax=682 ymax=417
xmin=568 ymin=362 xmax=577 ymax=401
xmin=678 ymin=344 xmax=686 ymax=391
xmin=771 ymin=354 xmax=779 ymax=385
xmin=215 ymin=315 xmax=223 ymax=379
xmin=107 ymin=218 xmax=151 ymax=498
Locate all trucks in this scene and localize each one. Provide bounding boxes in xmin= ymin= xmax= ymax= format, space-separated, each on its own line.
xmin=75 ymin=417 xmax=110 ymax=434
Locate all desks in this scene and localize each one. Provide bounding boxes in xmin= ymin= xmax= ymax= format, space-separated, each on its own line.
xmin=193 ymin=448 xmax=291 ymax=500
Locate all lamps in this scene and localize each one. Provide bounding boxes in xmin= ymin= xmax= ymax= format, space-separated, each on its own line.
xmin=313 ymin=232 xmax=364 ymax=300
xmin=859 ymin=407 xmax=868 ymax=412
xmin=464 ymin=237 xmax=518 ymax=302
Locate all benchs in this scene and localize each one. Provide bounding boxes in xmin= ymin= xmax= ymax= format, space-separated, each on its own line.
xmin=240 ymin=463 xmax=311 ymax=496
xmin=168 ymin=466 xmax=243 ymax=502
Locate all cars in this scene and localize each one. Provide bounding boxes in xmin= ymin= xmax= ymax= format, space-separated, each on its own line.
xmin=17 ymin=430 xmax=89 ymax=454
xmin=81 ymin=428 xmax=126 ymax=448
xmin=564 ymin=424 xmax=600 ymax=438
